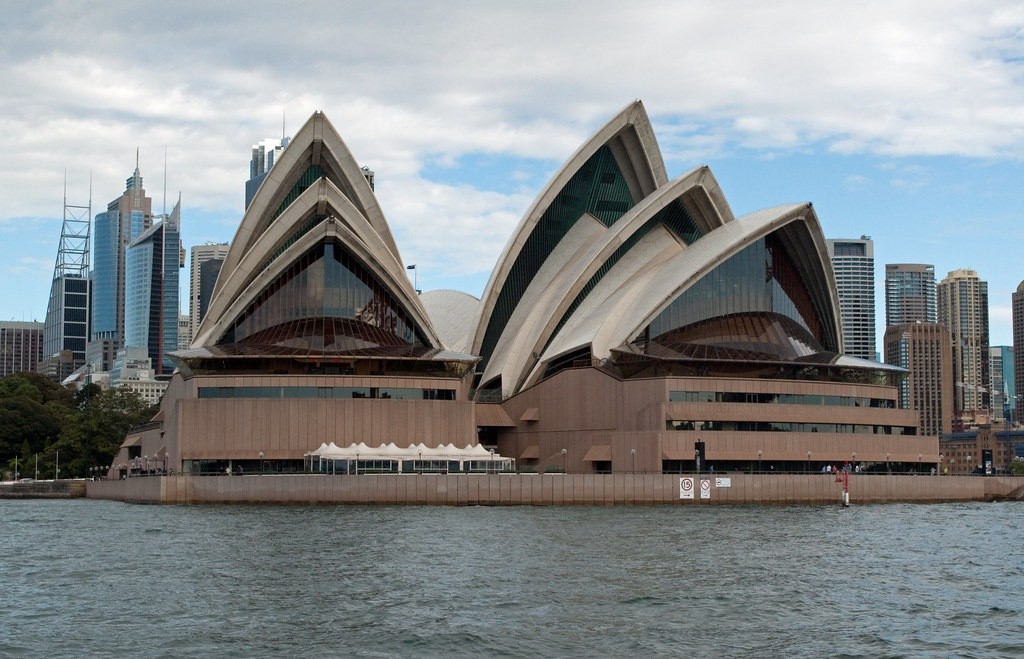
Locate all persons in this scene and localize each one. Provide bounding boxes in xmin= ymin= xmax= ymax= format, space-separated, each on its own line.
xmin=88 ymin=466 xmax=246 ymax=480
xmin=816 ymin=460 xmax=1013 ymax=475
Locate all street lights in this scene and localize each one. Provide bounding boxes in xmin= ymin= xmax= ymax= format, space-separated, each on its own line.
xmin=355 ymin=448 xmax=361 ymax=475
xmin=417 ymin=448 xmax=423 ymax=476
xmin=90 ymin=452 xmax=171 ymax=481
xmin=757 ymin=450 xmax=764 ymax=472
xmin=259 ymin=452 xmax=265 ymax=476
xmin=489 ymin=449 xmax=496 ymax=475
xmin=918 ymin=453 xmax=922 ymax=476
xmin=807 ymin=450 xmax=812 ymax=475
xmin=851 ymin=452 xmax=856 ymax=475
xmin=937 ymin=454 xmax=972 ymax=476
xmin=631 ymin=449 xmax=636 ymax=475
xmin=562 ymin=448 xmax=567 ymax=475
xmin=885 ymin=453 xmax=891 ymax=475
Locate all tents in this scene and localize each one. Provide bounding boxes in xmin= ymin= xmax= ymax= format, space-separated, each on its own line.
xmin=305 ymin=442 xmax=514 ymax=477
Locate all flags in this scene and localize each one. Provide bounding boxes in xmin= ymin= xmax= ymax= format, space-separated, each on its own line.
xmin=407 ymin=264 xmax=415 ymax=269
